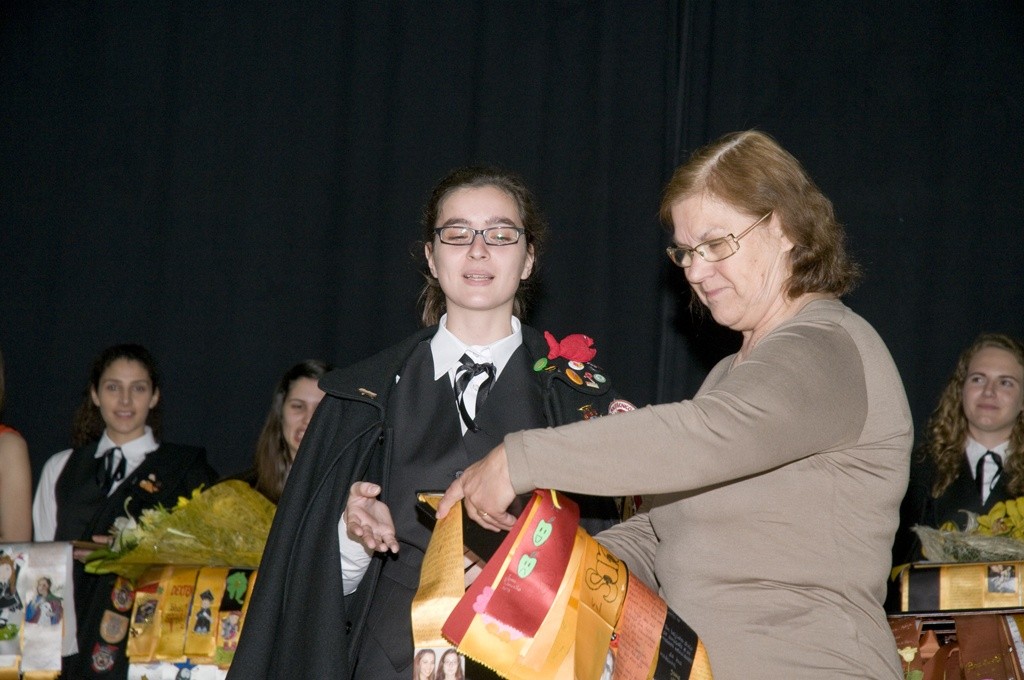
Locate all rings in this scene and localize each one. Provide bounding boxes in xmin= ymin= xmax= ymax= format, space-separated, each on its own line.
xmin=477 ymin=510 xmax=488 ymax=516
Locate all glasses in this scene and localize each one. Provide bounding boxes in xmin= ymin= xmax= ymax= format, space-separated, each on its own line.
xmin=666 ymin=210 xmax=774 ymax=268
xmin=429 ymin=226 xmax=528 ymax=247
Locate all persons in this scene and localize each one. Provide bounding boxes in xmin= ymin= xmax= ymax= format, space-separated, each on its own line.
xmin=0 ymin=359 xmax=32 ymax=541
xmin=413 ymin=649 xmax=436 ymax=680
xmin=434 ymin=130 xmax=916 ymax=680
xmin=435 ymin=648 xmax=464 ymax=680
xmin=215 ymin=360 xmax=330 ymax=505
xmin=31 ymin=343 xmax=220 ymax=680
xmin=227 ymin=165 xmax=623 ymax=680
xmin=883 ymin=332 xmax=1024 ymax=614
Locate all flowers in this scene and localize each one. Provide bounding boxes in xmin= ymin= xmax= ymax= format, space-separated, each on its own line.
xmin=84 ymin=482 xmax=204 ymax=574
